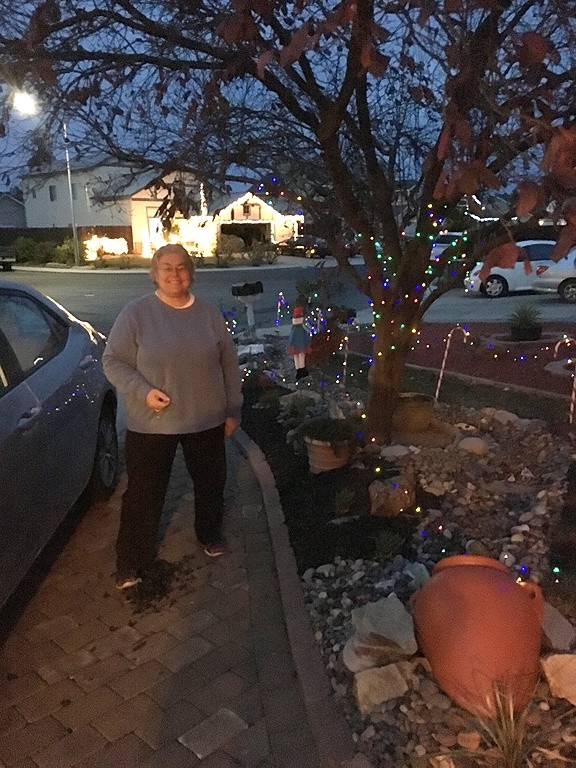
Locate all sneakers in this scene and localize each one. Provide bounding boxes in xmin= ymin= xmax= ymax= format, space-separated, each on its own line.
xmin=199 ymin=541 xmax=226 ymax=557
xmin=115 ymin=567 xmax=142 ymax=590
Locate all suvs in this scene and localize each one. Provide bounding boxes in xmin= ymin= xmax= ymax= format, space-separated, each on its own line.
xmin=464 ymin=240 xmax=558 ymax=298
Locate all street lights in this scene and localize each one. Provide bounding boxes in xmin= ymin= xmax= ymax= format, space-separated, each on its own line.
xmin=13 ymin=92 xmax=80 ymax=267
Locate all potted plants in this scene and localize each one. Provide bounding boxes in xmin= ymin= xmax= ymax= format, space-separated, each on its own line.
xmin=294 ymin=418 xmax=354 ymax=473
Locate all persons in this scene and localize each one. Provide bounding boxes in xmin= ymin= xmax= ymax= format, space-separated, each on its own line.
xmin=101 ymin=243 xmax=243 ymax=605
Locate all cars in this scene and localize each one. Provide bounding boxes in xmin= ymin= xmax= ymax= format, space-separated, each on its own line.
xmin=0 ymin=280 xmax=119 ymax=610
xmin=430 ymin=233 xmax=468 ymax=261
xmin=532 ymin=246 xmax=576 ymax=304
xmin=275 ymin=235 xmax=328 ymax=258
xmin=316 ymin=233 xmax=383 ymax=258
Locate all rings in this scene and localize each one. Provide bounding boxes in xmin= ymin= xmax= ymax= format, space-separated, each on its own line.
xmin=156 ymin=409 xmax=159 ymax=413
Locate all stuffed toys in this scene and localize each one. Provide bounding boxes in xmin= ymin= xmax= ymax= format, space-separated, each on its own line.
xmin=288 ymin=306 xmax=312 ymax=379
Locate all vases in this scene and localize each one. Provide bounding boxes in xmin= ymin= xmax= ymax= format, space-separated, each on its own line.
xmin=391 ymin=393 xmax=435 ymax=433
xmin=408 ymin=555 xmax=544 ymax=720
